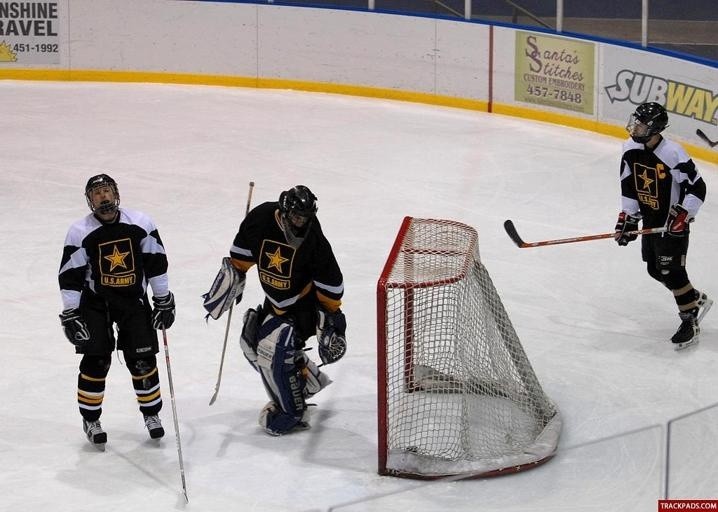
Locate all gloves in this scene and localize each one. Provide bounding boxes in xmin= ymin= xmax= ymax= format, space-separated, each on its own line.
xmin=151 ymin=291 xmax=175 ymax=330
xmin=59 ymin=307 xmax=97 ymax=346
xmin=666 ymin=204 xmax=695 ymax=242
xmin=614 ymin=212 xmax=644 ymax=246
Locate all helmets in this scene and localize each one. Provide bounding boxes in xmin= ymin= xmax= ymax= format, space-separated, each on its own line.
xmin=85 ymin=174 xmax=120 ymax=215
xmin=626 ymin=102 xmax=668 ymax=144
xmin=279 ymin=185 xmax=319 ymax=247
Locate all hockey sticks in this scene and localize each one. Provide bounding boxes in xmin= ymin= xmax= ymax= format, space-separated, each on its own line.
xmin=160 ymin=324 xmax=189 ymax=504
xmin=210 ymin=183 xmax=254 ymax=407
xmin=504 ymin=219 xmax=664 ymax=249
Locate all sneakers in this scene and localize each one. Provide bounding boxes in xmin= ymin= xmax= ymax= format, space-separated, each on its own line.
xmin=83 ymin=418 xmax=107 ymax=443
xmin=144 ymin=414 xmax=164 ymax=439
xmin=671 ymin=288 xmax=708 ymax=344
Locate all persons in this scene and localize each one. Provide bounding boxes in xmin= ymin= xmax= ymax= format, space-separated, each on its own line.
xmin=203 ymin=184 xmax=348 ymax=437
xmin=614 ymin=102 xmax=709 ymax=345
xmin=58 ymin=173 xmax=176 ymax=445
